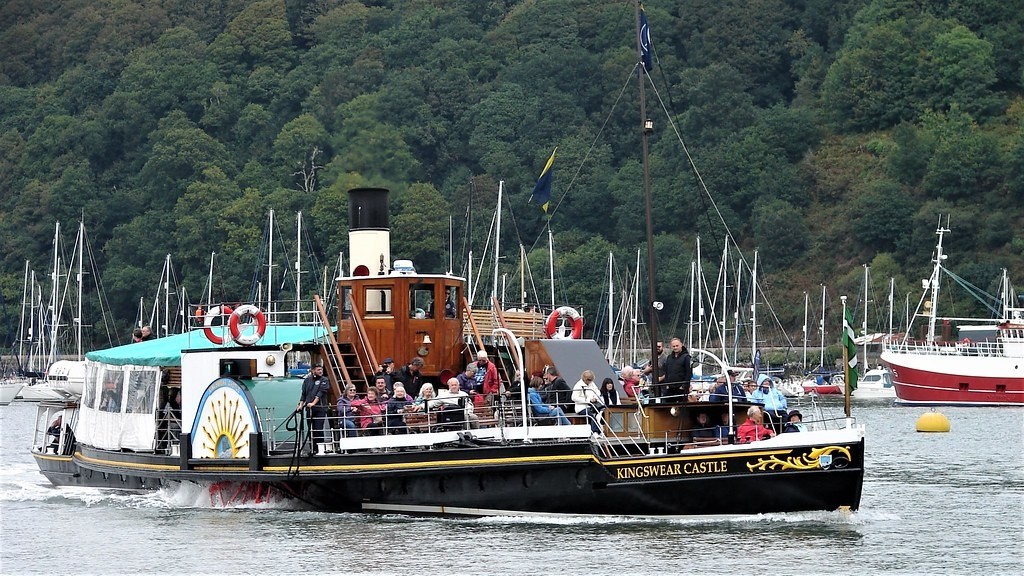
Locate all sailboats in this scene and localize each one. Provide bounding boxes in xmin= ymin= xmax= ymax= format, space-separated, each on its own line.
xmin=0 ymin=180 xmax=914 ymax=407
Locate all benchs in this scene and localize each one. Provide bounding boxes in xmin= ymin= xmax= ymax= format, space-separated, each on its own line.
xmin=463 ymin=308 xmax=548 ymax=339
xmin=403 ymin=400 xmax=499 ymax=433
xmin=693 ymin=436 xmax=736 ymax=448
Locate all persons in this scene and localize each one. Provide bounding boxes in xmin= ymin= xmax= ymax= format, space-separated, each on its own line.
xmin=600 ymin=378 xmax=622 ymax=405
xmin=494 ymin=368 xmax=573 ymax=427
xmin=429 ymin=287 xmax=455 ymax=319
xmin=297 ymin=364 xmax=330 ymax=454
xmin=100 ymin=381 xmax=181 ymax=452
xmin=621 ymin=337 xmax=807 ymax=445
xmin=48 ymin=415 xmax=76 ymax=455
xmin=337 ymin=357 xmax=478 ymax=452
xmin=132 ymin=326 xmax=153 ymax=343
xmin=571 ymin=370 xmax=605 ymax=433
xmin=473 ymin=350 xmax=498 ymax=428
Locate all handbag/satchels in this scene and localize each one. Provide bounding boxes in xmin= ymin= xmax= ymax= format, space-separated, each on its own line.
xmin=373 ymin=414 xmax=383 ymax=426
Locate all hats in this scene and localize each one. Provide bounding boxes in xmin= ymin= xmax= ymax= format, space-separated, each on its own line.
xmin=727 ymin=370 xmax=737 ymax=377
xmin=384 ymin=358 xmax=394 ymax=363
xmin=787 ymin=410 xmax=803 ymax=423
xmin=312 ymin=363 xmax=321 ymax=370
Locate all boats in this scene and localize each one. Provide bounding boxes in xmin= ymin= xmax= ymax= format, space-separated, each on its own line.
xmin=30 ymin=0 xmax=866 ymax=521
xmin=879 ymin=213 xmax=1024 ymax=406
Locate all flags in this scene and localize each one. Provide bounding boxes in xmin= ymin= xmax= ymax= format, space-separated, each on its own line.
xmin=636 ymin=9 xmax=652 ymax=78
xmin=532 ymin=152 xmax=555 ymax=214
xmin=841 ymin=301 xmax=858 ymax=396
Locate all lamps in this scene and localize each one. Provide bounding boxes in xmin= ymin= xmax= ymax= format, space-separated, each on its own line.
xmin=416 ymin=330 xmax=432 ymax=345
xmin=266 ymin=355 xmax=276 ymax=366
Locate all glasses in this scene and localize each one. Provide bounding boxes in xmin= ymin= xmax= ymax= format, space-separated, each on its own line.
xmin=478 ymin=359 xmax=486 ymax=362
xmin=699 ymin=391 xmax=705 ymax=394
xmin=348 ymin=389 xmax=356 ymax=392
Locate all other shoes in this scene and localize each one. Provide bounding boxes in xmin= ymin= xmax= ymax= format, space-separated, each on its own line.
xmin=591 ymin=432 xmax=602 ymax=439
xmin=308 ymin=450 xmax=316 ymax=456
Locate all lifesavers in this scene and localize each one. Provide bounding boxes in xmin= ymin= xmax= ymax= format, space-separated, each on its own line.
xmin=505 ymin=306 xmax=527 ymax=313
xmin=203 ymin=303 xmax=267 ymax=346
xmin=545 ymin=305 xmax=586 ymax=339
